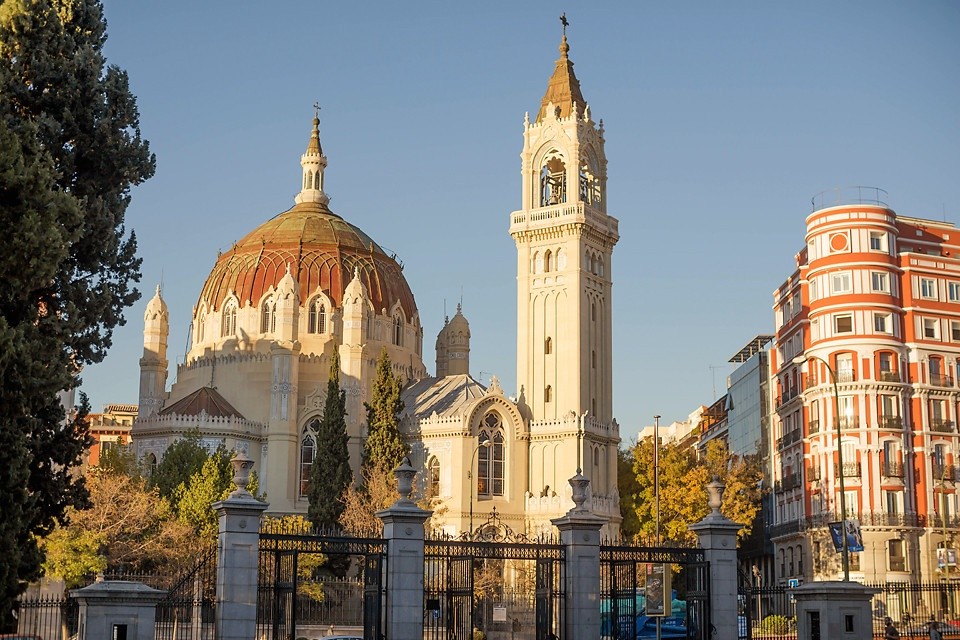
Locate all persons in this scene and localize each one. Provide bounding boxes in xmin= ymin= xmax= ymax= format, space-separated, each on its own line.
xmin=881 ymin=617 xmax=900 ymax=640
xmin=941 ymin=613 xmax=951 ymax=624
xmin=261 ymin=630 xmax=268 ymax=640
xmin=929 ymin=615 xmax=935 ymax=620
xmin=849 ymin=522 xmax=863 ymax=546
xmin=953 ymin=613 xmax=960 ymax=621
xmin=327 ymin=623 xmax=335 ymax=636
xmin=926 ymin=621 xmax=943 ymax=640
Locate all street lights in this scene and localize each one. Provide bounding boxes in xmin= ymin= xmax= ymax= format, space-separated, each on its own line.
xmin=468 ymin=440 xmax=492 ymax=556
xmin=792 ymin=356 xmax=851 ymax=581
xmin=653 ymin=414 xmax=661 ymax=546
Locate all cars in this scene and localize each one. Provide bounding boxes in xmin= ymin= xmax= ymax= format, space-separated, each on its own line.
xmin=630 ymin=601 xmax=695 ymax=640
xmin=475 ymin=600 xmax=524 ymax=632
xmin=900 ymin=621 xmax=958 ymax=633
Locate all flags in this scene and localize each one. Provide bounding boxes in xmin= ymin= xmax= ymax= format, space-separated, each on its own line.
xmin=845 ymin=519 xmax=865 ymax=551
xmin=828 ymin=522 xmax=844 ymax=553
